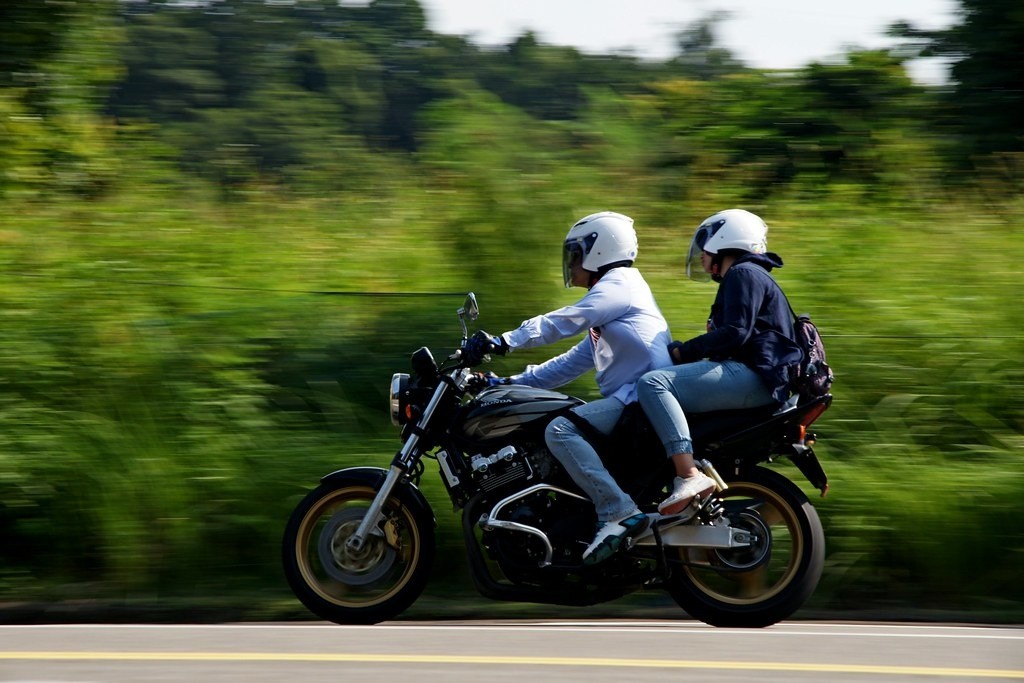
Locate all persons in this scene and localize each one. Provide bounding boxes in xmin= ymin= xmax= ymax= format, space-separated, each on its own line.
xmin=639 ymin=209 xmax=835 ymax=516
xmin=470 ymin=210 xmax=675 ymax=563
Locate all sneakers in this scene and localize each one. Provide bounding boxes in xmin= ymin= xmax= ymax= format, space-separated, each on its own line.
xmin=581 ymin=508 xmax=650 ymax=564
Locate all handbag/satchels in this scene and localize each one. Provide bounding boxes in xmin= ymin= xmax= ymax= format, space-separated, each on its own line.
xmin=793 ymin=314 xmax=832 ymax=400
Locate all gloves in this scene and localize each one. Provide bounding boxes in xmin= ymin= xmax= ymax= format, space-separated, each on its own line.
xmin=470 ymin=371 xmax=511 ymax=396
xmin=465 ymin=329 xmax=507 ymax=363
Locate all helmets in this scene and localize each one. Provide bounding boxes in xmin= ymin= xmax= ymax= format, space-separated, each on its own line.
xmin=565 ymin=211 xmax=639 ymax=272
xmin=696 ymin=208 xmax=768 ymax=255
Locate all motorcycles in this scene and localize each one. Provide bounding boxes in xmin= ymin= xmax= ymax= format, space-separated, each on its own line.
xmin=283 ymin=288 xmax=830 ymax=629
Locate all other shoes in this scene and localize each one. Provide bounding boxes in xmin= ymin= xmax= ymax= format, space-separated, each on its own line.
xmin=658 ymin=470 xmax=718 ymax=517
